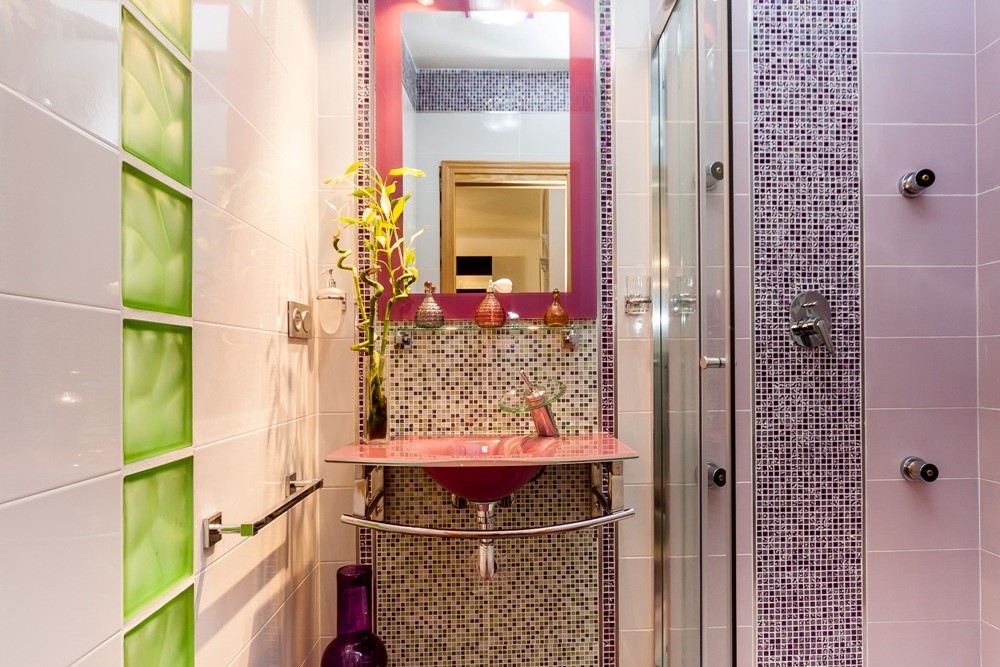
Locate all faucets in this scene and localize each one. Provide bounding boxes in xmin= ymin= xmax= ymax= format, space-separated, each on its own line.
xmin=519 ymin=369 xmax=559 ymax=436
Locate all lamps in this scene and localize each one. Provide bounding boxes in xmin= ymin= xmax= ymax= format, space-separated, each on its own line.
xmin=315 ymin=268 xmax=346 ymax=337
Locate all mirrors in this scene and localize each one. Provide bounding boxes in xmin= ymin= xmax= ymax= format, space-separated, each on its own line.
xmin=370 ymin=0 xmax=599 ymax=322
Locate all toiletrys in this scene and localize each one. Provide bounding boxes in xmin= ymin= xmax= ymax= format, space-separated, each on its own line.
xmin=318 ymin=267 xmax=344 ymax=333
xmin=544 ymin=289 xmax=570 ymax=327
xmin=476 ymin=279 xmax=506 ymax=327
xmin=415 ymin=280 xmax=444 ymax=328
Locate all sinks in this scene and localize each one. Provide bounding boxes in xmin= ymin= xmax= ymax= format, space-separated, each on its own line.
xmin=323 ymin=432 xmax=639 ymax=505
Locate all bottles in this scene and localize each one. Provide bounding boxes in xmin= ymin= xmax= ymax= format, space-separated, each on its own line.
xmin=320 ymin=565 xmax=387 ymax=667
